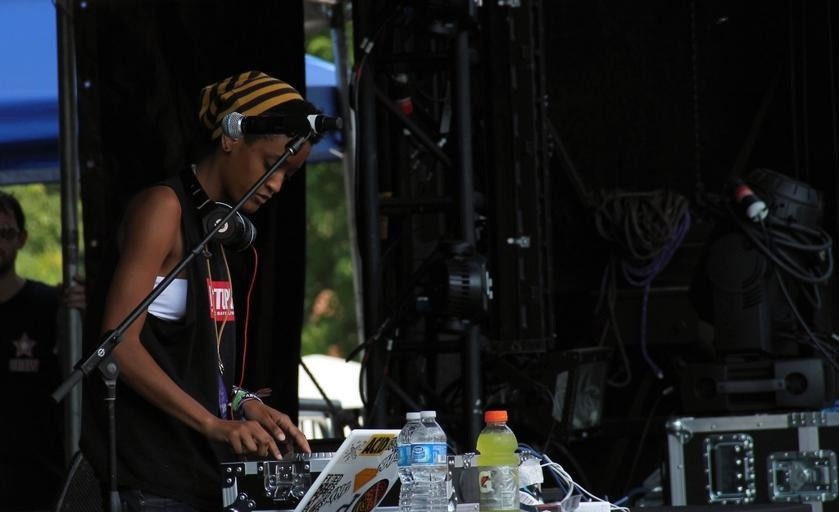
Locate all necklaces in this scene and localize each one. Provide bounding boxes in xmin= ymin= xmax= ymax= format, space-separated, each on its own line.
xmin=202 ymin=228 xmax=234 ymax=378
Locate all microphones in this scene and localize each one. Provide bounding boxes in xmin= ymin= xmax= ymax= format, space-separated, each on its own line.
xmin=391 ymin=70 xmax=415 ymax=119
xmin=221 ymin=111 xmax=344 ymax=139
xmin=726 ymin=173 xmax=770 ymax=223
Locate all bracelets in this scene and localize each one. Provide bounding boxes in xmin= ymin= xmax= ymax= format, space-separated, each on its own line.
xmin=228 ymin=383 xmax=265 ymax=413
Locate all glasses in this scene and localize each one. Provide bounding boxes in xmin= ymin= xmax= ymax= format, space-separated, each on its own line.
xmin=1 ymin=226 xmax=23 ymax=241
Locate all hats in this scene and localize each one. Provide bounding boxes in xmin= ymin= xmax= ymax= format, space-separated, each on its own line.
xmin=199 ymin=71 xmax=304 ymax=138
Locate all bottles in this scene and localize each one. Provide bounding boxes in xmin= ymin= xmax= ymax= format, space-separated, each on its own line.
xmin=395 ymin=413 xmax=429 ymax=512
xmin=409 ymin=410 xmax=449 ymax=512
xmin=475 ymin=411 xmax=521 ymax=512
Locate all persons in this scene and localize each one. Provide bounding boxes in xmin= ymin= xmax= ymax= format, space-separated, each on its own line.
xmin=97 ymin=70 xmax=328 ymax=512
xmin=299 ymin=288 xmax=347 ymax=358
xmin=0 ymin=190 xmax=88 ymax=510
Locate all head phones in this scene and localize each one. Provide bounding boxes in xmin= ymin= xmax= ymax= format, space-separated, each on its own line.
xmin=178 ymin=160 xmax=258 ymax=254
